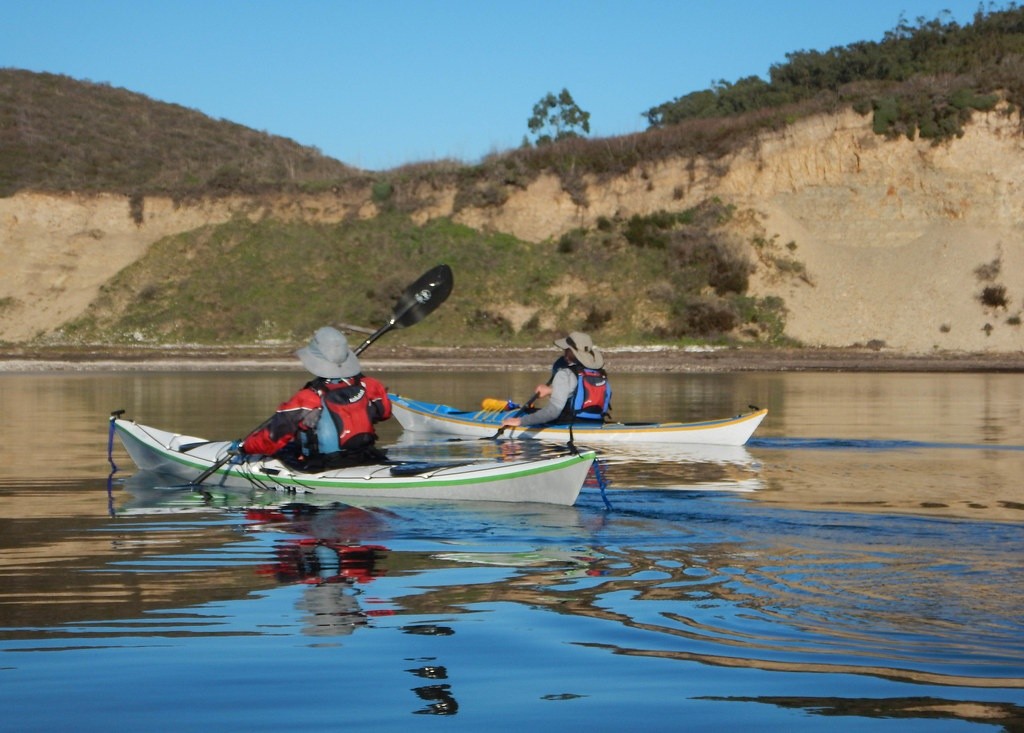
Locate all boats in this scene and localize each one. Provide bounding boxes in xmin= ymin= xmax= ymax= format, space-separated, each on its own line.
xmin=386 ymin=392 xmax=769 ymax=446
xmin=106 ymin=407 xmax=597 ymax=506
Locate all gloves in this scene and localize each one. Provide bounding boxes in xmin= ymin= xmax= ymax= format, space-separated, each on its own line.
xmin=226 ymin=438 xmax=244 ymax=456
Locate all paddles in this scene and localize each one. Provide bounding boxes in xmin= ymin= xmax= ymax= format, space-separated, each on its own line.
xmin=188 ymin=263 xmax=457 ymax=487
xmin=489 ymin=355 xmax=569 ymax=440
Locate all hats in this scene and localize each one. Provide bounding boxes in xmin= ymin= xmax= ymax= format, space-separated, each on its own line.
xmin=552 ymin=331 xmax=604 ymax=370
xmin=295 ymin=327 xmax=362 ymax=379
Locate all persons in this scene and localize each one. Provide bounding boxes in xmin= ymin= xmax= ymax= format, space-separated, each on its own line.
xmin=240 ymin=503 xmax=402 ymax=640
xmin=226 ymin=326 xmax=392 ymax=467
xmin=502 ymin=332 xmax=612 ymax=428
